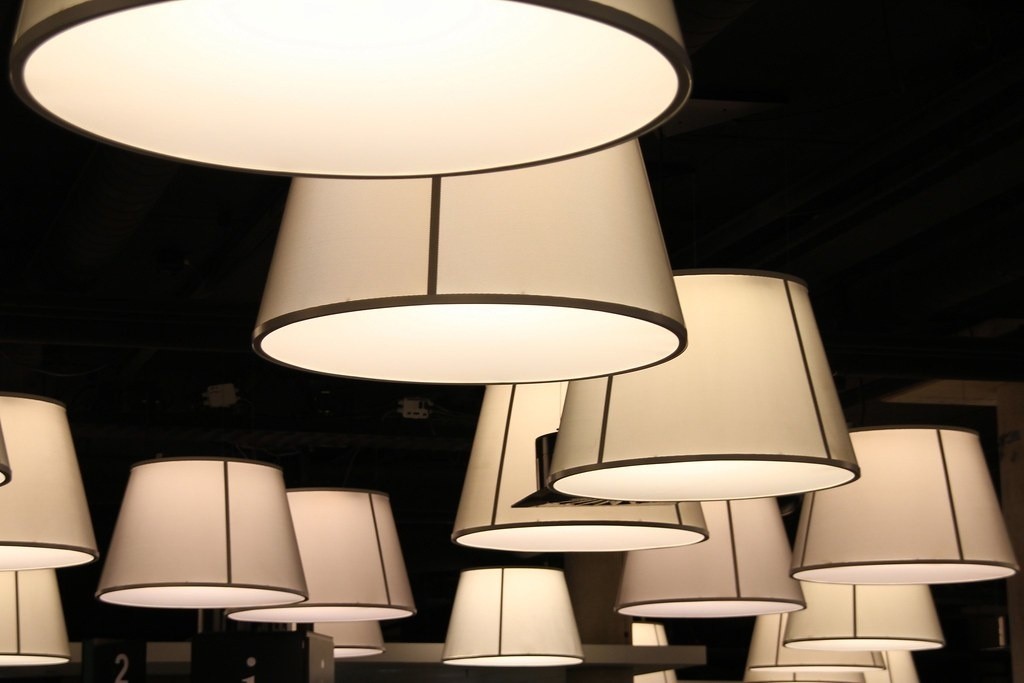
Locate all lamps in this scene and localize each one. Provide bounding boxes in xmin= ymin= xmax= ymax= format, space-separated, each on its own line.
xmin=450 ymin=373 xmax=710 ymax=554
xmin=0 ymin=391 xmax=100 ymax=572
xmin=288 ymin=623 xmax=385 ymax=658
xmin=790 ymin=422 xmax=1021 ymax=584
xmin=741 ymin=613 xmax=921 ymax=683
xmin=785 ymin=580 xmax=946 ymax=653
xmin=0 ymin=422 xmax=12 ymax=487
xmin=11 ymin=0 xmax=693 ymax=178
xmin=0 ymin=568 xmax=72 ymax=667
xmin=631 ymin=621 xmax=679 ymax=683
xmin=95 ymin=458 xmax=310 ymax=610
xmin=252 ymin=138 xmax=687 ymax=387
xmin=222 ymin=486 xmax=416 ymax=622
xmin=614 ymin=496 xmax=804 ymax=619
xmin=546 ymin=268 xmax=862 ymax=501
xmin=441 ymin=568 xmax=583 ymax=668
